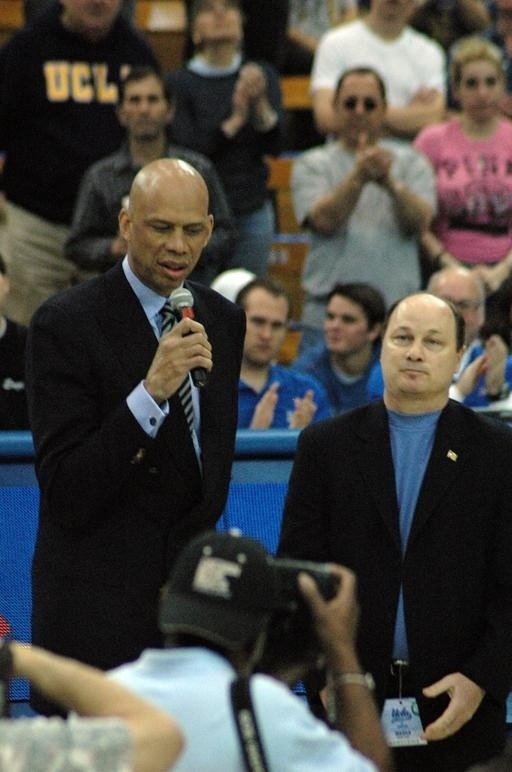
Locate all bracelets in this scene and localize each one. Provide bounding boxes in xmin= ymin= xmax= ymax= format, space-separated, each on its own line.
xmin=328 ymin=672 xmax=378 ymax=691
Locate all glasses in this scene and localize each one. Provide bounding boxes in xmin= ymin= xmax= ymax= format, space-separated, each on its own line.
xmin=344 ymin=96 xmax=375 ymax=111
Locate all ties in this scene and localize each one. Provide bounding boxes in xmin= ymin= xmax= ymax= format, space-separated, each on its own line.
xmin=159 ymin=301 xmax=194 ymax=440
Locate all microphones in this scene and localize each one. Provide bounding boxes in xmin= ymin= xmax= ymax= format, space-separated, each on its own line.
xmin=168 ymin=288 xmax=208 ymax=388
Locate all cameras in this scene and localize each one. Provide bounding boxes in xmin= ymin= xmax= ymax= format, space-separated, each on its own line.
xmin=263 ymin=562 xmax=335 ymax=656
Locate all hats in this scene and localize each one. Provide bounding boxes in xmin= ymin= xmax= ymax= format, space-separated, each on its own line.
xmin=157 ymin=526 xmax=275 ymax=650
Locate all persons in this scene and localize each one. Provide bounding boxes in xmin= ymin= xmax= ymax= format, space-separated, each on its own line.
xmin=267 ymin=290 xmax=511 ymax=770
xmin=1 ymin=0 xmax=512 ymax=436
xmin=22 ymin=155 xmax=244 ymax=714
xmin=58 ymin=530 xmax=401 ymax=771
xmin=1 ymin=633 xmax=187 ymax=771
